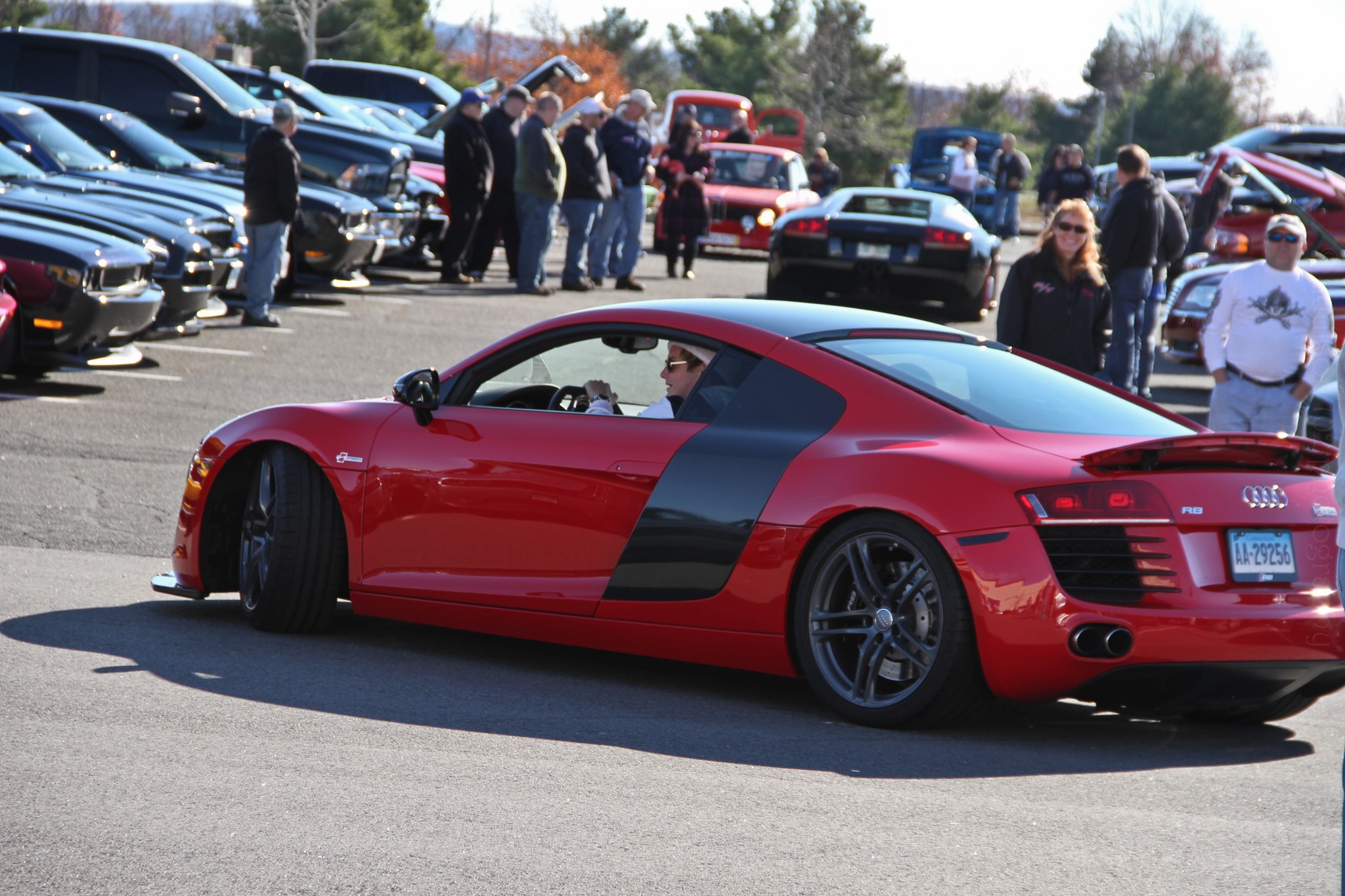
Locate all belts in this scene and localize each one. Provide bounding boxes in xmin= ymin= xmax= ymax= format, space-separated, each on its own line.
xmin=1226 ymin=361 xmax=1306 ymax=387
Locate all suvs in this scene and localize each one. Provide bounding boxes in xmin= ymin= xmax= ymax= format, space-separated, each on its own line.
xmin=0 ymin=92 xmax=379 ymax=383
xmin=1091 ymin=122 xmax=1345 ymax=230
xmin=0 ymin=23 xmax=416 ymax=256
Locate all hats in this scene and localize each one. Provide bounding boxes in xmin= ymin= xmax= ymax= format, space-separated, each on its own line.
xmin=1265 ymin=213 xmax=1307 ymax=241
xmin=581 ymin=97 xmax=614 ymax=116
xmin=630 ymin=89 xmax=658 ymax=110
xmin=682 ymin=103 xmax=698 ymax=115
xmin=505 ymin=84 xmax=536 ymax=104
xmin=273 ymin=98 xmax=304 ymax=122
xmin=459 ymin=86 xmax=491 ymax=105
xmin=668 ymin=340 xmax=717 ymax=366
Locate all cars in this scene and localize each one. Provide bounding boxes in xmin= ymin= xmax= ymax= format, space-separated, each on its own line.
xmin=651 ymin=144 xmax=816 ymax=250
xmin=891 ymin=127 xmax=1003 ymax=232
xmin=1159 ymin=145 xmax=1345 ymax=366
xmin=211 ymin=55 xmax=590 ymax=244
xmin=665 ymin=90 xmax=807 ymax=156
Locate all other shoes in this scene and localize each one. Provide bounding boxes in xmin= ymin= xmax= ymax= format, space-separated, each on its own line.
xmin=241 ymin=310 xmax=281 ymax=327
xmin=517 ymin=282 xmax=557 ymax=296
xmin=683 ymin=266 xmax=693 ymax=278
xmin=562 ymin=277 xmax=595 ymax=291
xmin=615 ymin=273 xmax=643 ymax=291
xmin=591 ymin=276 xmax=603 ymax=286
xmin=440 ymin=272 xmax=474 ymax=284
xmin=667 ymin=264 xmax=677 ymax=278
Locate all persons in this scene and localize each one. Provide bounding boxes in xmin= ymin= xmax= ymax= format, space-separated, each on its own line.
xmin=656 ymin=120 xmax=716 ymax=277
xmin=804 ymin=147 xmax=841 ymax=194
xmin=1140 ymin=179 xmax=1188 ymax=397
xmin=561 ymin=98 xmax=612 ymax=290
xmin=667 ymin=103 xmax=698 ymax=144
xmin=585 ymin=339 xmax=717 ymax=418
xmin=1202 ymin=213 xmax=1339 ymax=436
xmin=1098 ymin=144 xmax=1166 ymax=395
xmin=243 ymin=99 xmax=302 ymax=326
xmin=588 ymin=88 xmax=658 ymax=291
xmin=950 ymin=136 xmax=979 ymax=215
xmin=1047 ymin=142 xmax=1095 ymax=215
xmin=725 ymin=108 xmax=751 ymax=143
xmin=985 ymin=132 xmax=1032 ymax=240
xmin=441 ymin=87 xmax=494 ymax=284
xmin=513 ymin=90 xmax=567 ymax=294
xmin=999 ymin=197 xmax=1112 ymax=375
xmin=1037 ymin=143 xmax=1064 ymax=228
xmin=464 ymin=83 xmax=534 ymax=281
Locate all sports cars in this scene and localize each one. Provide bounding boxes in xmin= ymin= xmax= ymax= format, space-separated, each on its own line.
xmin=764 ymin=185 xmax=1005 ymax=319
xmin=153 ymin=298 xmax=1344 ymax=732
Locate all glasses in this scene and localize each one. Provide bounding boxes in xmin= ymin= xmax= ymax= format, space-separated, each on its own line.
xmin=598 ymin=114 xmax=605 ymax=118
xmin=1266 ymin=232 xmax=1300 ymax=243
xmin=665 ymin=358 xmax=688 ymax=373
xmin=472 ymin=101 xmax=482 ymax=108
xmin=1056 ymin=221 xmax=1088 ymax=234
xmin=688 ymin=132 xmax=701 ymax=137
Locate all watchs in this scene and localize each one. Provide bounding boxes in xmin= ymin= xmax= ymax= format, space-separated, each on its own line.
xmin=590 ymin=393 xmax=612 ymax=404
